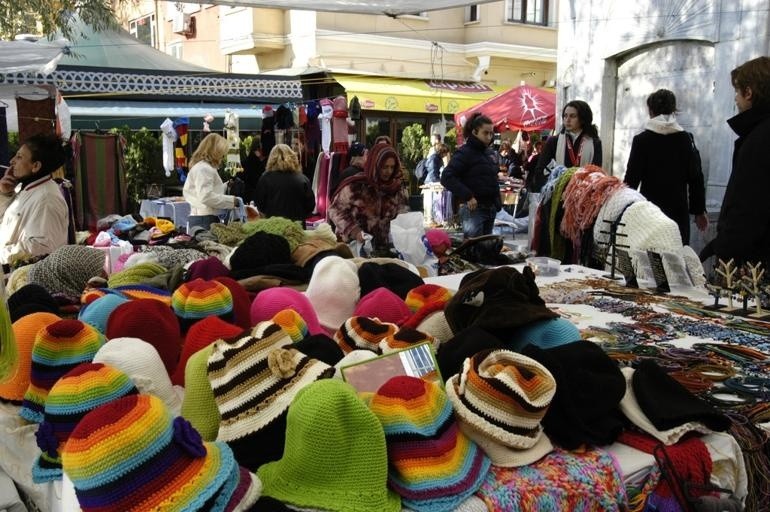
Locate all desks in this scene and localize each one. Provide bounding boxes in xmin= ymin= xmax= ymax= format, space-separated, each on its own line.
xmin=139 ymin=198 xmax=241 ymax=229
xmin=418 ymin=183 xmax=525 ymax=230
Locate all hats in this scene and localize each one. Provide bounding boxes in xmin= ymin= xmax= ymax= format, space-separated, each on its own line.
xmin=262 ymin=96 xmax=361 ymax=128
xmin=159 ymin=111 xmax=238 ymax=159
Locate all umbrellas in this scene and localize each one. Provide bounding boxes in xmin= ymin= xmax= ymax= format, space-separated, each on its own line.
xmin=454 ymin=80 xmax=556 ymax=131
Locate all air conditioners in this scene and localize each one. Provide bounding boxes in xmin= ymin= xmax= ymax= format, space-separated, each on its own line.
xmin=174 ymin=13 xmax=191 ymax=34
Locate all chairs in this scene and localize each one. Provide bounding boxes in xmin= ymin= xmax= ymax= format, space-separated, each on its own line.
xmin=493 ymin=193 xmax=519 ymax=240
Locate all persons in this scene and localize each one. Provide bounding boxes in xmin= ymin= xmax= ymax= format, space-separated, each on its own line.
xmin=181 ymin=133 xmax=552 ymax=246
xmin=440 ymin=112 xmax=502 ymax=238
xmin=623 ymin=89 xmax=710 ymax=246
xmin=533 ymin=101 xmax=602 ymax=269
xmin=698 ymin=56 xmax=770 ymax=273
xmin=0 ymin=131 xmax=74 ymax=283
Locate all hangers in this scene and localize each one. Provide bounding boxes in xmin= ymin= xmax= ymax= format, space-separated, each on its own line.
xmin=80 ymin=120 xmax=117 ymax=138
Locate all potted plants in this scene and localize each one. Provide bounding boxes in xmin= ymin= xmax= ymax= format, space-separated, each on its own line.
xmin=401 ymin=121 xmax=432 ymax=212
